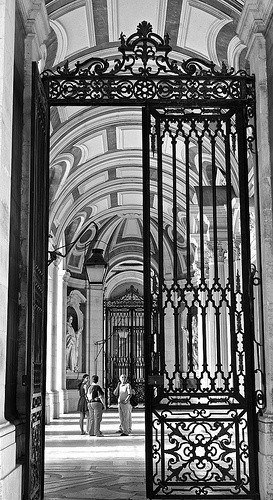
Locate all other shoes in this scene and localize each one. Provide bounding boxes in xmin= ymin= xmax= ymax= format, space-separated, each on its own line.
xmin=80 ymin=431 xmax=88 ymax=435
xmin=90 ymin=432 xmax=104 ymax=437
xmin=120 ymin=433 xmax=128 ymax=436
xmin=116 ymin=430 xmax=122 ymax=433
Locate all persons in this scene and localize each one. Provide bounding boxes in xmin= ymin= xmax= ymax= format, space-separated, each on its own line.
xmin=77 ymin=374 xmax=91 ymax=435
xmin=86 ymin=375 xmax=106 ymax=437
xmin=66 ymin=315 xmax=83 ymax=372
xmin=113 ymin=374 xmax=135 ymax=437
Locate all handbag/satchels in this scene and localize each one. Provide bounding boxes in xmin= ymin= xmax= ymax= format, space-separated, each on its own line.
xmin=130 ymin=394 xmax=139 ymax=406
